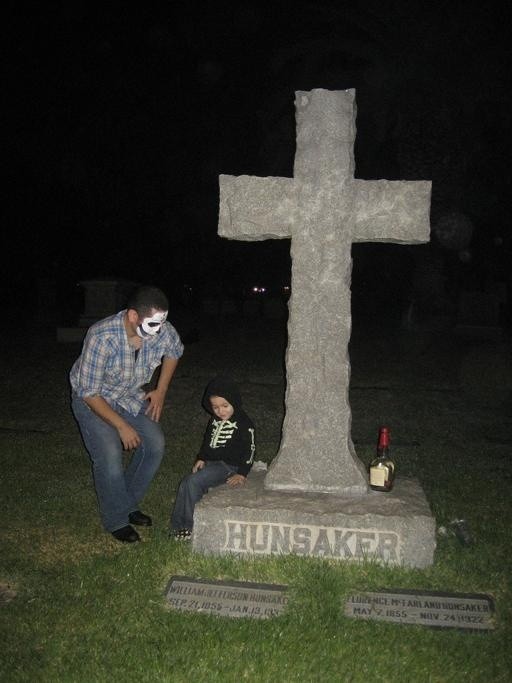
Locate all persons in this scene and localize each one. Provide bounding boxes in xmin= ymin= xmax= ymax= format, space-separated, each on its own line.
xmin=170 ymin=374 xmax=257 ymax=540
xmin=68 ymin=286 xmax=185 ymax=545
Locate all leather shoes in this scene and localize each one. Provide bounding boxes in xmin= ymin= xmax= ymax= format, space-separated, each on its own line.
xmin=129 ymin=510 xmax=153 ymax=526
xmin=111 ymin=525 xmax=140 ymax=542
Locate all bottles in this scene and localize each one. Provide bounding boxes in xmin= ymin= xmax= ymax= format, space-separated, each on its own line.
xmin=368 ymin=424 xmax=396 ymax=492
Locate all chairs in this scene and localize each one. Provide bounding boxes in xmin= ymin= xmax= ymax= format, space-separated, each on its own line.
xmin=56 ymin=270 xmax=131 ymax=377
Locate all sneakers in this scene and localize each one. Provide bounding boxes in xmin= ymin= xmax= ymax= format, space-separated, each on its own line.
xmin=169 ymin=529 xmax=191 ymax=541
